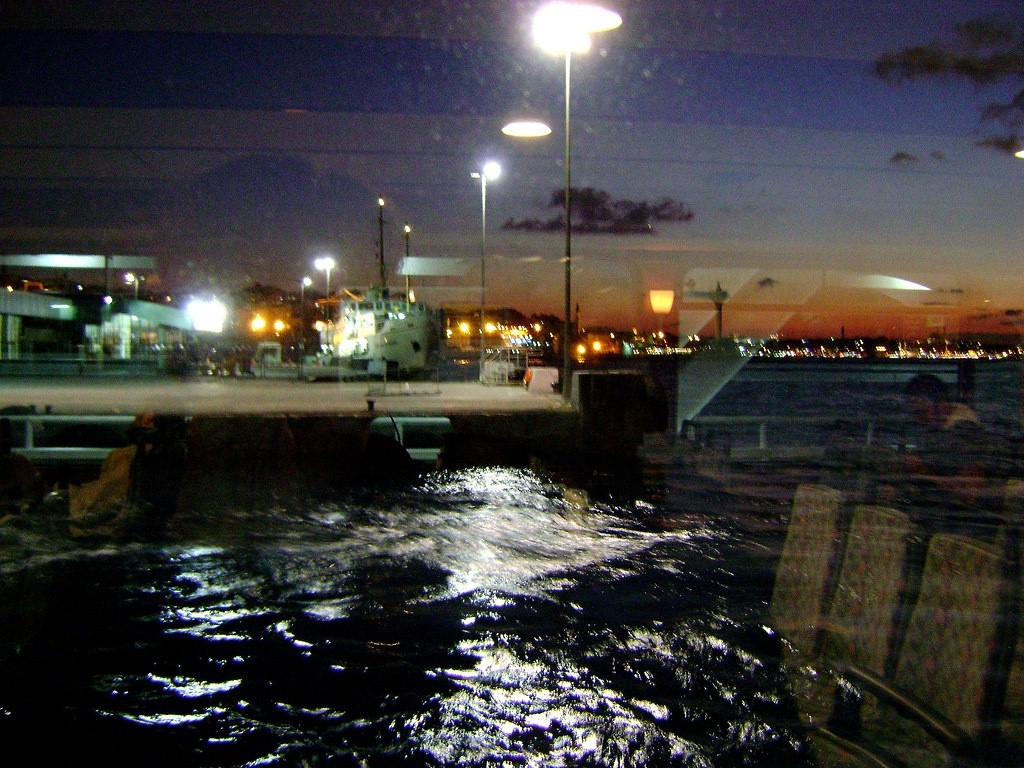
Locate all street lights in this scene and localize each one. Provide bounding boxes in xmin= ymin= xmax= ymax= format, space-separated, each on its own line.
xmin=478 ymin=159 xmax=501 ymax=383
xmin=404 ymin=220 xmax=410 ymax=314
xmin=533 ymin=2 xmax=622 ymax=405
xmin=378 ymin=193 xmax=386 ymax=302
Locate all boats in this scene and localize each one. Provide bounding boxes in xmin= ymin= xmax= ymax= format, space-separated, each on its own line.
xmin=297 ymin=286 xmax=438 ymax=382
